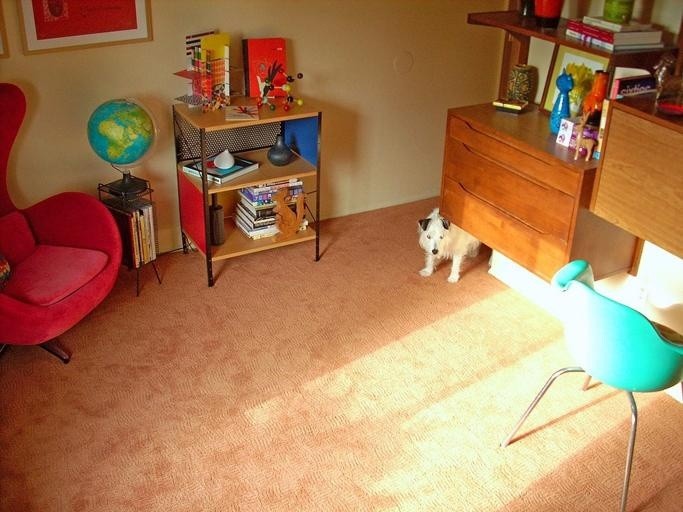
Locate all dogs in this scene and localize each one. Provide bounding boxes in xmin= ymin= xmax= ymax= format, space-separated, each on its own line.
xmin=417 ymin=207 xmax=481 ymax=284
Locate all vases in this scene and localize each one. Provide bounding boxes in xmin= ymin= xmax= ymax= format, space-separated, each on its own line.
xmin=506 ymin=64 xmax=533 ymax=102
xmin=267 ymin=134 xmax=292 ymax=166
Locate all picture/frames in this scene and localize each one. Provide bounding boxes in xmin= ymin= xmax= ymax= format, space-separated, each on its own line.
xmin=0 ymin=0 xmax=10 ymax=59
xmin=16 ymin=0 xmax=153 ymax=56
xmin=539 ymin=44 xmax=612 ymax=117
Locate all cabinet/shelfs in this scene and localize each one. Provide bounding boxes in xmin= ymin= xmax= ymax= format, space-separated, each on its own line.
xmin=98 ymin=176 xmax=162 ymax=297
xmin=440 ymin=0 xmax=683 ymax=284
xmin=173 ymin=93 xmax=322 ymax=287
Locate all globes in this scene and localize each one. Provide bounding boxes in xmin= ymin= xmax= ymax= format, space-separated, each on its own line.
xmin=86 ymin=96 xmax=158 ymax=195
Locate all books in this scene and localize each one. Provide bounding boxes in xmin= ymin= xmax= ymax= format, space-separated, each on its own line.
xmin=607 ymin=74 xmax=655 ymax=99
xmin=100 ymin=195 xmax=157 ymax=270
xmin=563 ymin=27 xmax=665 ymax=53
xmin=593 ymin=89 xmax=659 ymax=161
xmin=224 ymin=105 xmax=259 ymax=122
xmin=581 ymin=14 xmax=653 ymax=33
xmin=566 ymin=16 xmax=662 ymax=47
xmin=240 ymin=37 xmax=288 ymax=99
xmin=181 ymin=150 xmax=259 ymax=185
xmin=231 ymin=177 xmax=308 ymax=241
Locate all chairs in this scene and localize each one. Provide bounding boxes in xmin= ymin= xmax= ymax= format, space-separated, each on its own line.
xmin=503 ymin=260 xmax=683 ymax=512
xmin=0 ymin=83 xmax=124 ymax=364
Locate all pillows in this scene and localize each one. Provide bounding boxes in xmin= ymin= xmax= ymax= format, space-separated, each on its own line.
xmin=0 ymin=212 xmax=37 ymax=266
xmin=0 ymin=255 xmax=10 ymax=292
